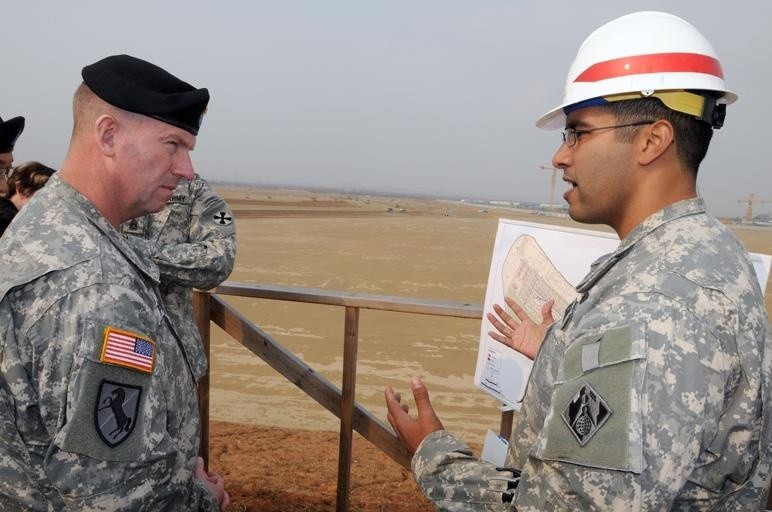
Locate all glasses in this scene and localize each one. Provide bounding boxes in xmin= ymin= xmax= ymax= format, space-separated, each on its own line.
xmin=1 ymin=164 xmax=15 ymax=179
xmin=561 ymin=119 xmax=674 ymax=148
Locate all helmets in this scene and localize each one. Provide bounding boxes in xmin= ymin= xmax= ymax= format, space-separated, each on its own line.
xmin=537 ymin=12 xmax=738 ymax=132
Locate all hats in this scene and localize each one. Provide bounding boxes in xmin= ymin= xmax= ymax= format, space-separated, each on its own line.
xmin=0 ymin=115 xmax=24 ymax=153
xmin=82 ymin=54 xmax=209 ymax=136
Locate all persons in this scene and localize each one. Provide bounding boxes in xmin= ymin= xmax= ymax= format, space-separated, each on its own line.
xmin=0 ymin=54 xmax=232 ymax=511
xmin=8 ymin=160 xmax=57 ymax=214
xmin=117 ymin=168 xmax=234 ymax=384
xmin=387 ymin=13 xmax=770 ymax=510
xmin=2 ymin=116 xmax=24 ymax=237
